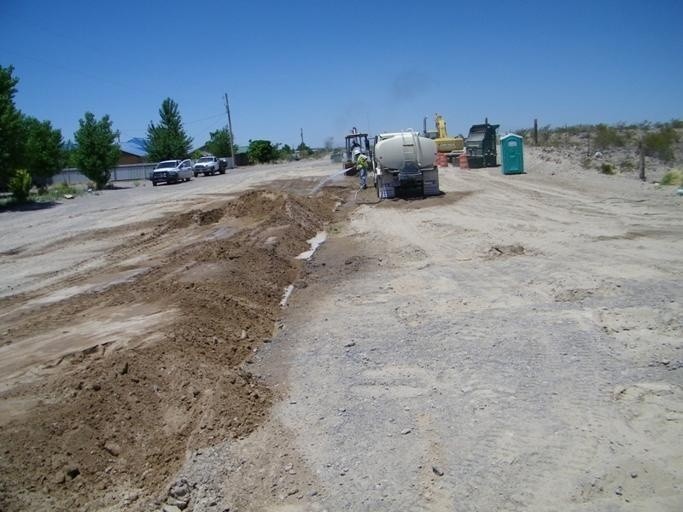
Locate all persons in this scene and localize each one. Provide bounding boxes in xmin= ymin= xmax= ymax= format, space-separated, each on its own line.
xmin=353 ymin=150 xmax=368 ymax=190
xmin=350 ymin=140 xmax=360 ymax=151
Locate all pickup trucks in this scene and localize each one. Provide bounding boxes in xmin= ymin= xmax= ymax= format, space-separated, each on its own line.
xmin=194 ymin=155 xmax=226 ymax=178
xmin=150 ymin=158 xmax=191 ymax=185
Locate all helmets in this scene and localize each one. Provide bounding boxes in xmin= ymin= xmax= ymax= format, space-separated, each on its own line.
xmin=355 ymin=150 xmax=360 ymax=156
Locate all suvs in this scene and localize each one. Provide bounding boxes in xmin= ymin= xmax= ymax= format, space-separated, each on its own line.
xmin=291 ymin=151 xmax=301 ymax=160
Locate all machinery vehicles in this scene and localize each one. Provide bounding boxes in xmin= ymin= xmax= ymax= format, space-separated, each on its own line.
xmin=424 ymin=111 xmax=464 ymax=167
xmin=370 ymin=127 xmax=440 ymax=198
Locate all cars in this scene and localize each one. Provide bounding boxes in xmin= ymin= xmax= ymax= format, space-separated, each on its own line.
xmin=342 ymin=126 xmax=373 ymax=175
